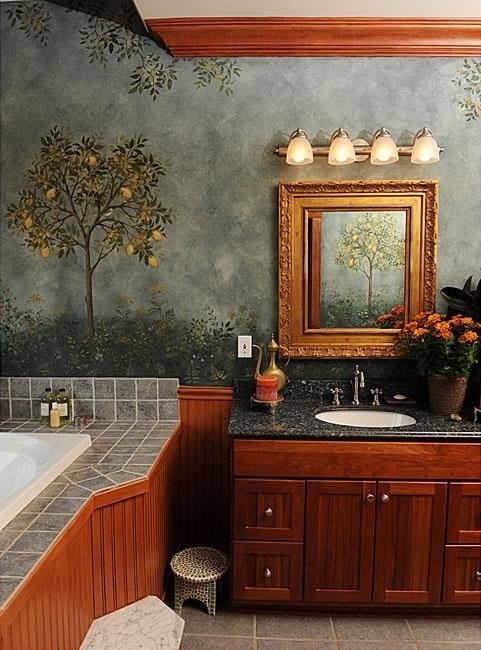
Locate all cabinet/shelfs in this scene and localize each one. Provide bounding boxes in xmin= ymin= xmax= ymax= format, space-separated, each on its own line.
xmin=442 ymin=480 xmax=481 ymax=614
xmin=303 ymin=479 xmax=448 ymax=614
xmin=233 ymin=475 xmax=305 ymax=608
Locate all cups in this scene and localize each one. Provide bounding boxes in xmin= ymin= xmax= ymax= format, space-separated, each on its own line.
xmin=255 ymin=376 xmax=277 ymax=400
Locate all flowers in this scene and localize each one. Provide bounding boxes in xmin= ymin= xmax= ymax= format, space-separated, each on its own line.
xmin=392 ymin=309 xmax=481 ymax=381
xmin=375 ymin=303 xmax=403 ymax=328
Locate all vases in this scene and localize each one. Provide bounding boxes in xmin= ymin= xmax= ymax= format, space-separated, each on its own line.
xmin=427 ymin=373 xmax=469 ymax=416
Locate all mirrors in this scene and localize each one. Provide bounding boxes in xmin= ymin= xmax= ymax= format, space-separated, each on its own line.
xmin=278 ymin=178 xmax=440 ymax=361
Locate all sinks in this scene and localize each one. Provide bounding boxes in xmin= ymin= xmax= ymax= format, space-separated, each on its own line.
xmin=314 ymin=410 xmax=416 ymax=428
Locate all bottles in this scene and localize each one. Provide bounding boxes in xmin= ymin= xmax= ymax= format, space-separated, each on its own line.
xmin=48 ymin=401 xmax=62 ymax=429
xmin=39 ymin=387 xmax=70 ymax=423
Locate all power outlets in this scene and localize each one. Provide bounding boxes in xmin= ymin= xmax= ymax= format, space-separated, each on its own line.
xmin=238 ymin=334 xmax=254 ymax=359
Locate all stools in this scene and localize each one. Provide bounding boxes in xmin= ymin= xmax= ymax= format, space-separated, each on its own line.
xmin=169 ymin=546 xmax=230 ymax=618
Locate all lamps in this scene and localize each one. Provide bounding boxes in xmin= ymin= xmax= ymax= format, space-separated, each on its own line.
xmin=271 ymin=126 xmax=446 ymax=166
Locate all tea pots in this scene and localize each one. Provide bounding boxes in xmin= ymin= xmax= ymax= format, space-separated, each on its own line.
xmin=250 ymin=332 xmax=290 ymax=393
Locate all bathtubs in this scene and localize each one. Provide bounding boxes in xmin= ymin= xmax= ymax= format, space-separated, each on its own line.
xmin=0 ymin=429 xmax=94 ymax=534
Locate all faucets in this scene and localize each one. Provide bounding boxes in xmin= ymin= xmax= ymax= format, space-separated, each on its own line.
xmin=351 ymin=363 xmax=367 ymax=405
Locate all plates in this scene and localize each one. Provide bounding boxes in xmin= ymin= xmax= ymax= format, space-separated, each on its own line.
xmin=251 ymin=394 xmax=286 ymax=404
